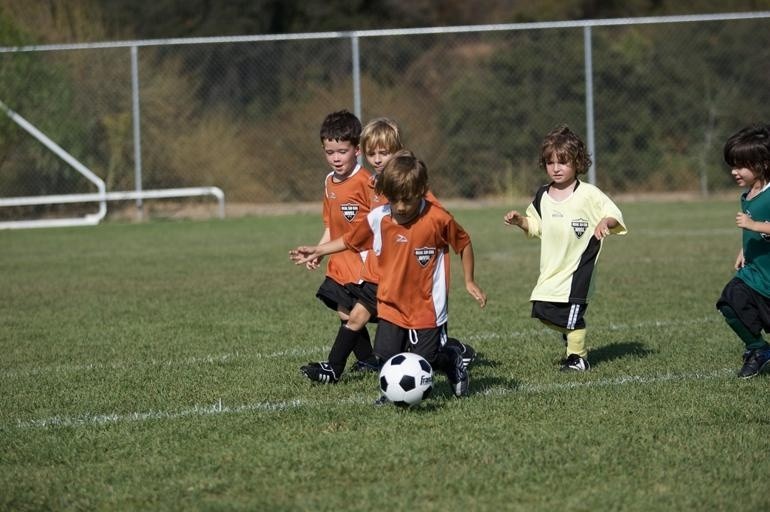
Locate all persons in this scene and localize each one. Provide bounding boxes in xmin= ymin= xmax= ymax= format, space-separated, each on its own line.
xmin=307 ymin=108 xmax=378 ymax=376
xmin=716 ymin=122 xmax=770 ymax=378
xmin=503 ymin=123 xmax=628 ymax=372
xmin=288 ymin=149 xmax=486 ymax=408
xmin=300 ymin=117 xmax=477 ymax=383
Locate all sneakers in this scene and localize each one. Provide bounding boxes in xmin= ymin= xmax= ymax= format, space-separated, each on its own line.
xmin=445 ymin=346 xmax=469 ymax=398
xmin=558 ymin=354 xmax=591 ymax=373
xmin=738 ymin=341 xmax=767 ymax=379
xmin=349 ymin=361 xmax=380 ymax=374
xmin=300 ymin=359 xmax=341 ymax=384
xmin=460 ymin=341 xmax=477 ymax=369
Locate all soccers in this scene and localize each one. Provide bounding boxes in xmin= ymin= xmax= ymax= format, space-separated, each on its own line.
xmin=378 ymin=352 xmax=434 ymax=407
